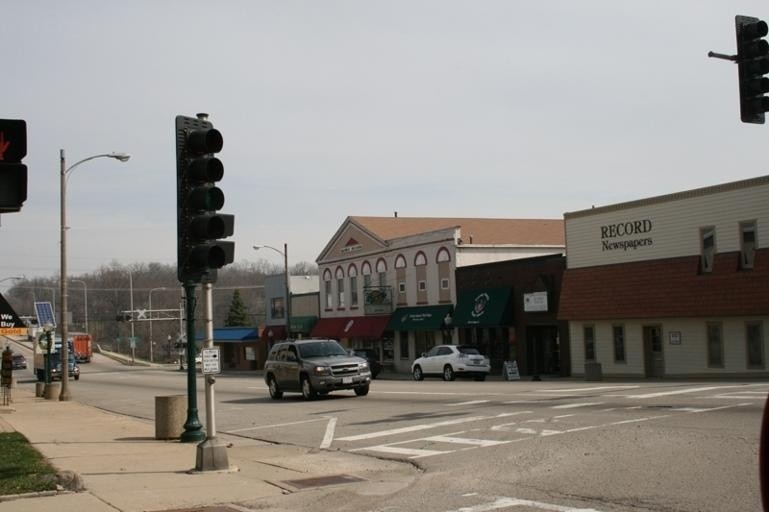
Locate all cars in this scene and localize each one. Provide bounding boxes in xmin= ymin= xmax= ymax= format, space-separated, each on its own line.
xmin=346 ymin=348 xmax=379 ymax=377
xmin=10 ymin=354 xmax=28 ymax=369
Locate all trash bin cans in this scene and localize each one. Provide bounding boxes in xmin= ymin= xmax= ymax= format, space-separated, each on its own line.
xmin=47 ymin=384 xmax=58 ymax=399
xmin=37 ymin=383 xmax=45 ymax=396
xmin=584 ymin=363 xmax=602 ymax=381
xmin=155 ymin=396 xmax=187 ymax=439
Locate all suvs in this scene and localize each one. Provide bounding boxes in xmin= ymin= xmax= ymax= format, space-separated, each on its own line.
xmin=411 ymin=343 xmax=491 ymax=382
xmin=263 ymin=339 xmax=371 ymax=399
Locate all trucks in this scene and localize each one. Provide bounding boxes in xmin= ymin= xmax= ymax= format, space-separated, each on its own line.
xmin=67 ymin=332 xmax=93 ymax=363
xmin=34 ymin=332 xmax=81 ymax=381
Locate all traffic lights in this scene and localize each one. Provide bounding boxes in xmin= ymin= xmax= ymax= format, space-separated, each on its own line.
xmin=217 ymin=213 xmax=234 ymax=264
xmin=735 ymin=15 xmax=769 ymax=124
xmin=0 ymin=118 xmax=28 ymax=212
xmin=176 ymin=115 xmax=223 ymax=282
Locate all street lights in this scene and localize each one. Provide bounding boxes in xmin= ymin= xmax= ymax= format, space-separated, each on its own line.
xmin=70 ymin=280 xmax=88 ymax=333
xmin=253 ymin=242 xmax=292 ymax=336
xmin=168 ymin=335 xmax=172 ymax=362
xmin=0 ymin=275 xmax=24 ymax=282
xmin=59 ymin=148 xmax=132 ymax=400
xmin=149 ymin=286 xmax=166 ymax=362
